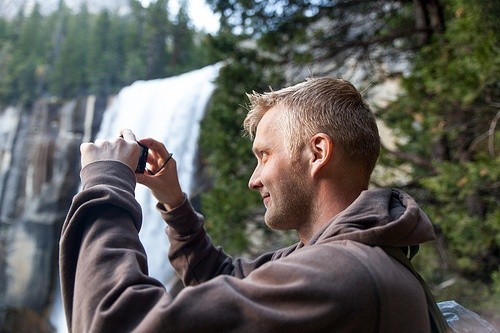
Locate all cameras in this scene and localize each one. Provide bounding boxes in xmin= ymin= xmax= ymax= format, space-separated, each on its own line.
xmin=119 ymin=135 xmax=148 ymax=174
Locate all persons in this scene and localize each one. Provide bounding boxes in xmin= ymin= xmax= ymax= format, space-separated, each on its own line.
xmin=58 ymin=77 xmax=451 ymax=333
